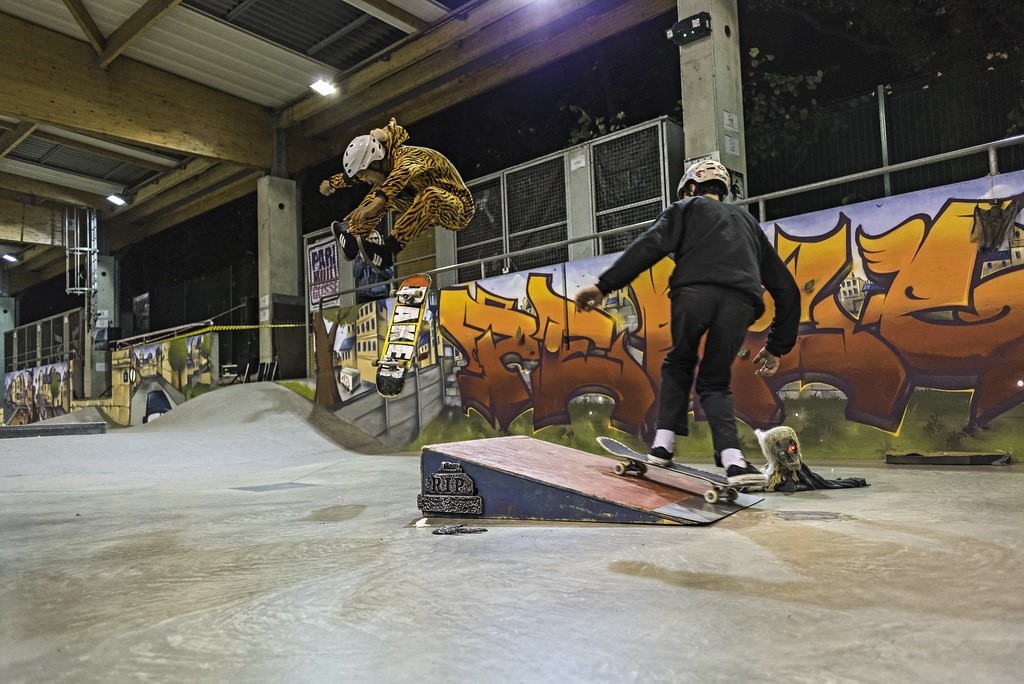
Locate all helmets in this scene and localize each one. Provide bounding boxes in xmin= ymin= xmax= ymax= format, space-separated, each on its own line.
xmin=676 ymin=159 xmax=732 ymax=202
xmin=342 ymin=134 xmax=385 ymax=177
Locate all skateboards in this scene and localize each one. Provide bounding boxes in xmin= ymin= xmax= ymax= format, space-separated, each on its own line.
xmin=372 ymin=273 xmax=432 ymax=399
xmin=595 ymin=435 xmax=770 ymax=505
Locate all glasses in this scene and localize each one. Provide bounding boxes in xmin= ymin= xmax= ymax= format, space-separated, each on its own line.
xmin=369 ymin=237 xmax=379 ymax=240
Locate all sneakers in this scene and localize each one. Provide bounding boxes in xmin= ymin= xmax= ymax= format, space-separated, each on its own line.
xmin=647 ymin=445 xmax=674 ymax=466
xmin=726 ymin=460 xmax=767 ymax=484
xmin=331 ymin=220 xmax=358 ymax=262
xmin=356 ymin=236 xmax=394 ymax=272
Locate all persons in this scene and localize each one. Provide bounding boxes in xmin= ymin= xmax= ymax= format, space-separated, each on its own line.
xmin=576 ymin=161 xmax=803 ymax=487
xmin=353 ymin=230 xmax=396 ymax=306
xmin=318 ymin=119 xmax=473 ymax=276
